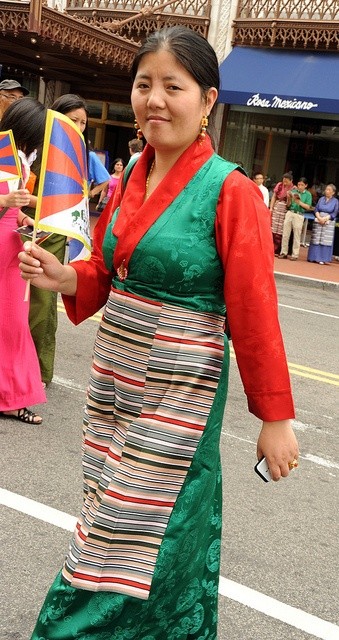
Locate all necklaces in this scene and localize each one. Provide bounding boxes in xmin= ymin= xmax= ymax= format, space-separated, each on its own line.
xmin=146 ymin=161 xmax=154 ymax=201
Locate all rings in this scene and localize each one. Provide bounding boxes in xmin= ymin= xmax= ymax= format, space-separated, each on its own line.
xmin=290 ymin=459 xmax=299 ymax=470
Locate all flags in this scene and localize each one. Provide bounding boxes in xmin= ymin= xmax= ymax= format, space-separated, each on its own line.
xmin=0 ymin=129 xmax=22 ymax=182
xmin=35 ymin=108 xmax=92 ymax=263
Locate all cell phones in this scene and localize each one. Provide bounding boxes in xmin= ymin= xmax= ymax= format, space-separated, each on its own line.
xmin=251 ymin=453 xmax=276 ymax=486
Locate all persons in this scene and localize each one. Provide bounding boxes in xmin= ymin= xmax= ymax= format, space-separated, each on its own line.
xmin=21 ymin=93 xmax=88 ymax=389
xmin=17 ymin=25 xmax=299 ymax=640
xmin=252 ymin=171 xmax=269 ymax=208
xmin=96 ymin=158 xmax=125 ymax=211
xmin=278 ymin=177 xmax=312 ymax=261
xmin=0 ymin=97 xmax=47 ymax=425
xmin=0 ymin=79 xmax=29 ymax=122
xmin=88 ymin=150 xmax=112 ymax=202
xmin=269 ymin=173 xmax=297 ymax=257
xmin=307 ymin=184 xmax=338 ymax=265
xmin=235 ymin=161 xmax=243 ymax=168
xmin=128 ymin=139 xmax=144 ymax=158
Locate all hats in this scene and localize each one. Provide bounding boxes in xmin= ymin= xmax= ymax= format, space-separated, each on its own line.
xmin=0 ymin=79 xmax=30 ymax=96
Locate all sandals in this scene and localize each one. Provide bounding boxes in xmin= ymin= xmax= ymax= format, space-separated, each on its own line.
xmin=0 ymin=406 xmax=44 ymax=426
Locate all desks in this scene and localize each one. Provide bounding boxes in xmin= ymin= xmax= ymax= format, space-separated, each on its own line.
xmin=300 ymin=212 xmax=315 ymax=247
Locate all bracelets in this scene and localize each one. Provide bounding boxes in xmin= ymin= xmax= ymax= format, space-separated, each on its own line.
xmin=21 ymin=216 xmax=27 ymax=227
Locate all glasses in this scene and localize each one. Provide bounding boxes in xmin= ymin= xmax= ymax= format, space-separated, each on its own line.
xmin=1 ymin=94 xmax=21 ymax=102
